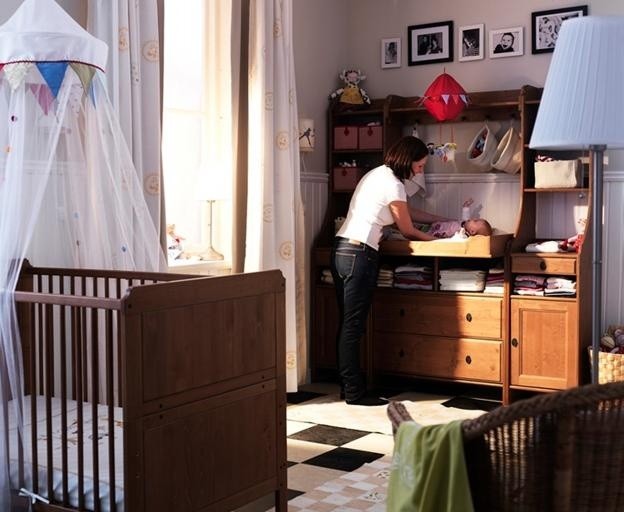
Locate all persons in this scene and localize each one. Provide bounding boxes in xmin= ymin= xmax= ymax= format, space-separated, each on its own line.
xmin=426 ymin=39 xmax=441 ymax=54
xmin=419 ymin=37 xmax=431 ymax=55
xmin=329 ymin=135 xmax=459 ymax=405
xmin=493 ymin=33 xmax=515 ymax=53
xmin=392 ymin=197 xmax=492 ymax=239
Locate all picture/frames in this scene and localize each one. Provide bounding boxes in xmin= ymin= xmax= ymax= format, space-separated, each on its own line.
xmin=488 ymin=25 xmax=523 ymax=58
xmin=381 ymin=36 xmax=401 ymax=69
xmin=531 ymin=4 xmax=588 ymax=53
xmin=458 ymin=23 xmax=484 ymax=62
xmin=407 ymin=19 xmax=453 ymax=67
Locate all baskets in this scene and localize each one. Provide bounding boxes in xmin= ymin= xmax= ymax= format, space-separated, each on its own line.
xmin=587 ymin=345 xmax=624 ymax=385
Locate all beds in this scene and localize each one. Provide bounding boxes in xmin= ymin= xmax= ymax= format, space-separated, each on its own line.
xmin=1 ymin=256 xmax=284 ymax=510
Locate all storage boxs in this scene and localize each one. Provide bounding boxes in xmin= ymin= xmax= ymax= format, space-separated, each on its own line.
xmin=332 ymin=163 xmax=374 ymax=191
xmin=534 ymin=157 xmax=587 ymax=190
xmin=356 ymin=126 xmax=384 ymax=151
xmin=332 ymin=124 xmax=358 ymax=150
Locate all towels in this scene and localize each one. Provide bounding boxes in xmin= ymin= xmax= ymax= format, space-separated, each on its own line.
xmin=380 ymin=418 xmax=497 ymax=511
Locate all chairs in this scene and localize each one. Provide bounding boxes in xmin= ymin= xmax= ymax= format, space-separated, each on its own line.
xmin=382 ymin=371 xmax=624 ymax=512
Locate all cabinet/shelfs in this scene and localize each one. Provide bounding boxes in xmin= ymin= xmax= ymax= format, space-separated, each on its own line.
xmin=505 ymin=254 xmax=592 ymax=409
xmin=366 ymin=243 xmax=510 ymax=410
xmin=308 ymin=246 xmax=371 ymax=382
xmin=510 ymin=80 xmax=606 ymax=252
xmin=320 ymin=90 xmax=402 ymax=246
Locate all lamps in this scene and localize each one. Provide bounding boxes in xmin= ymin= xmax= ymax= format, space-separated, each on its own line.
xmin=528 ymin=13 xmax=623 ymax=399
xmin=298 ymin=118 xmax=316 ymax=175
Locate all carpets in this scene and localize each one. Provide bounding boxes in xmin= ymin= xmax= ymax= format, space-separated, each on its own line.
xmin=275 ymin=450 xmax=408 ymax=512
xmin=284 ymin=390 xmax=488 ymax=434
